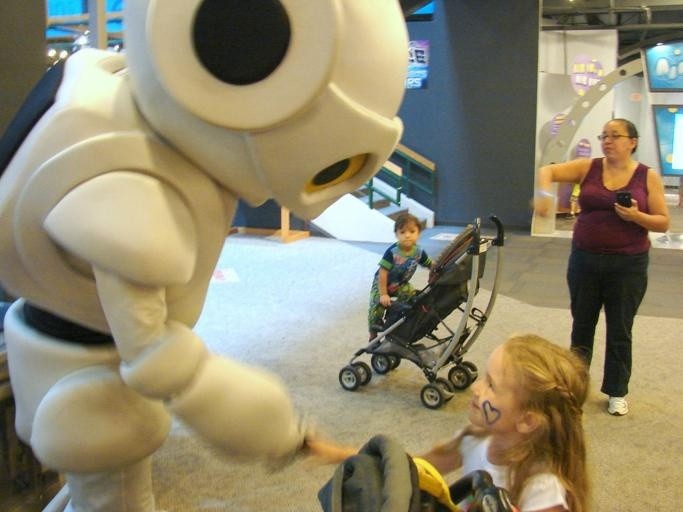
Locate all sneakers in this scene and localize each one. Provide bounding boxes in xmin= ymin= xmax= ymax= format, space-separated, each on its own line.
xmin=607 ymin=395 xmax=629 ymax=416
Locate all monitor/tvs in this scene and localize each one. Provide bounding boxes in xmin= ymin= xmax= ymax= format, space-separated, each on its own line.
xmin=645 ymin=38 xmax=683 ymax=92
xmin=652 ymin=104 xmax=683 ymax=177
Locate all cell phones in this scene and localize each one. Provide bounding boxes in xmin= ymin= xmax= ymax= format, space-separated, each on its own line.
xmin=617 ymin=192 xmax=631 ymax=208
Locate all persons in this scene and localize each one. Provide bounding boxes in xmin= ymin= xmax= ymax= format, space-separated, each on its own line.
xmin=366 ymin=211 xmax=439 ymax=345
xmin=0 ymin=0 xmax=416 ymax=512
xmin=304 ymin=331 xmax=592 ymax=511
xmin=530 ymin=114 xmax=671 ymax=420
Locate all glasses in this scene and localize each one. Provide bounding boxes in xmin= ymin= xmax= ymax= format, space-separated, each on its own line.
xmin=597 ymin=133 xmax=634 ymax=140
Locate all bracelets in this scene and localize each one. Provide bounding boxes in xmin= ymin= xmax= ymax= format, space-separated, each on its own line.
xmin=538 ymin=190 xmax=556 ymax=200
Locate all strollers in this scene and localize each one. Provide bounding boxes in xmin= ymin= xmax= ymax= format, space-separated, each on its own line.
xmin=340 ymin=215 xmax=505 ymax=409
